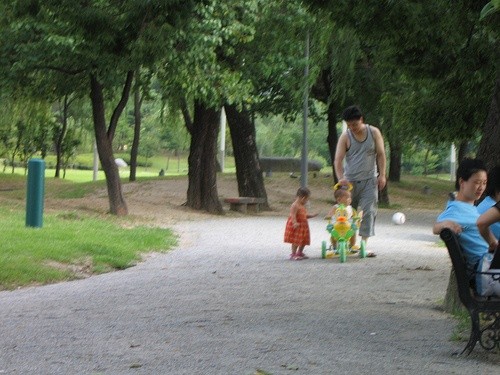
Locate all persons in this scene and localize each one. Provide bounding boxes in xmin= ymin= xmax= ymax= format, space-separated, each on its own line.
xmin=333 ymin=106 xmax=387 ymax=258
xmin=283 ymin=187 xmax=319 ymax=262
xmin=432 ymin=159 xmax=500 ymax=295
xmin=329 ymin=189 xmax=358 ymax=254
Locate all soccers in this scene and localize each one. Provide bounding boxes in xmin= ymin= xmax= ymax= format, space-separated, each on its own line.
xmin=392 ymin=212 xmax=406 ymax=225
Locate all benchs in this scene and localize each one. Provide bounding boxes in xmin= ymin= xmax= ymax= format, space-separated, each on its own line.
xmin=440 ymin=228 xmax=500 ymax=358
xmin=223 ymin=197 xmax=266 ymax=213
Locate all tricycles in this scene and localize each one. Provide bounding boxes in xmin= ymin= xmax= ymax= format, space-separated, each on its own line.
xmin=321 ymin=182 xmax=373 ymax=263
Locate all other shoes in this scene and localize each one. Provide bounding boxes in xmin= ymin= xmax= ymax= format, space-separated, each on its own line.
xmin=298 ymin=252 xmax=308 ymax=259
xmin=291 ymin=254 xmax=302 ymax=260
xmin=365 ymin=250 xmax=375 ymax=257
